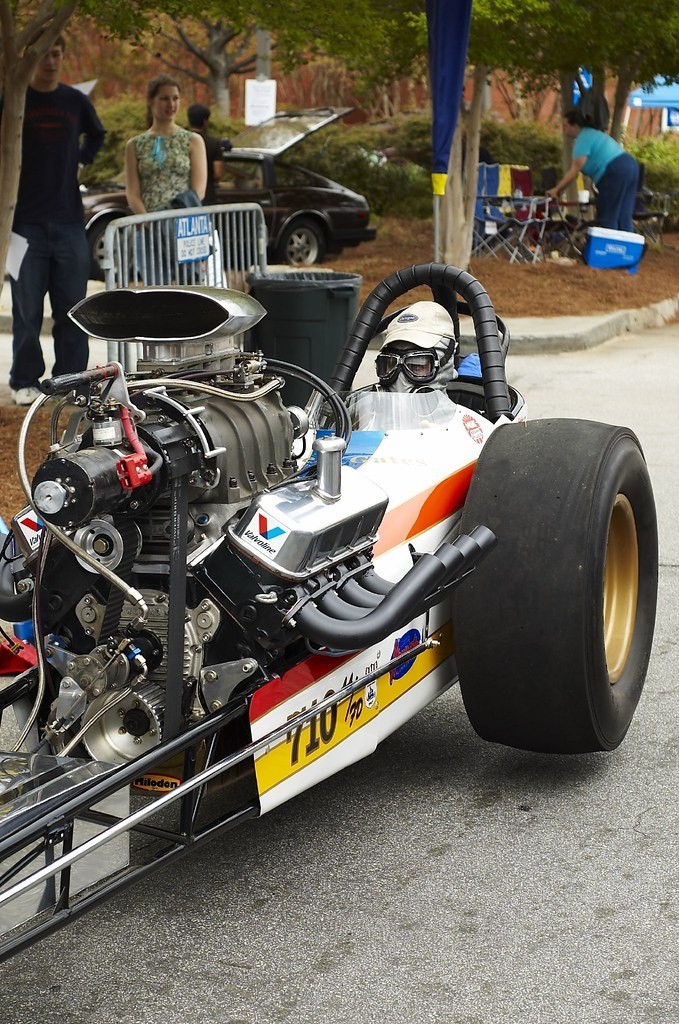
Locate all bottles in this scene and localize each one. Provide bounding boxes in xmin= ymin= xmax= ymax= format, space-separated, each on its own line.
xmin=514 ymin=188 xmax=528 ymax=211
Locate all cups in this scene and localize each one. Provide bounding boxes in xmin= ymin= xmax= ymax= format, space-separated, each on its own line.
xmin=579 ymin=189 xmax=589 ymax=212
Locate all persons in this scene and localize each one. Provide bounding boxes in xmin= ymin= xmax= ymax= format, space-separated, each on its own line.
xmin=124 ymin=74 xmax=229 ymax=290
xmin=548 ymin=111 xmax=639 ymax=234
xmin=285 ymin=300 xmax=497 ymax=511
xmin=0 ymin=28 xmax=106 ymax=406
xmin=184 ymin=101 xmax=233 ymax=205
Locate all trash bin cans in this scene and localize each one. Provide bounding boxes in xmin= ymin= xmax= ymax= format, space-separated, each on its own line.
xmin=247 ymin=273 xmax=363 ymax=410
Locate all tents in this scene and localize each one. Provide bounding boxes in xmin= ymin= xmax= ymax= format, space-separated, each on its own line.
xmin=624 ymin=71 xmax=679 ymax=137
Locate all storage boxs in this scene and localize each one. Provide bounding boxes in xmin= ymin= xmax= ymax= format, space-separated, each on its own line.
xmin=584 ymin=225 xmax=645 ymax=275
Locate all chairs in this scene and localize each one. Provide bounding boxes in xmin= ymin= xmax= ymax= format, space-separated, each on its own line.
xmin=589 ymin=162 xmax=669 ymax=244
xmin=471 ymin=161 xmax=584 ymax=266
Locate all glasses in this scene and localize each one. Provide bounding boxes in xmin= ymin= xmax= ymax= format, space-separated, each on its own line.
xmin=374 ymin=339 xmax=455 ymax=387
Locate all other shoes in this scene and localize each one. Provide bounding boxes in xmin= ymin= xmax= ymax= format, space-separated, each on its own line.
xmin=11 ymin=387 xmax=40 ymax=405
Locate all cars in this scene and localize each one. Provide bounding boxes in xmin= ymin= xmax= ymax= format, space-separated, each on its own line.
xmin=76 ymin=107 xmax=378 ymax=281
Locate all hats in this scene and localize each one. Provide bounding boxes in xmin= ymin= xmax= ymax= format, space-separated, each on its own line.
xmin=380 ymin=301 xmax=454 ymax=348
